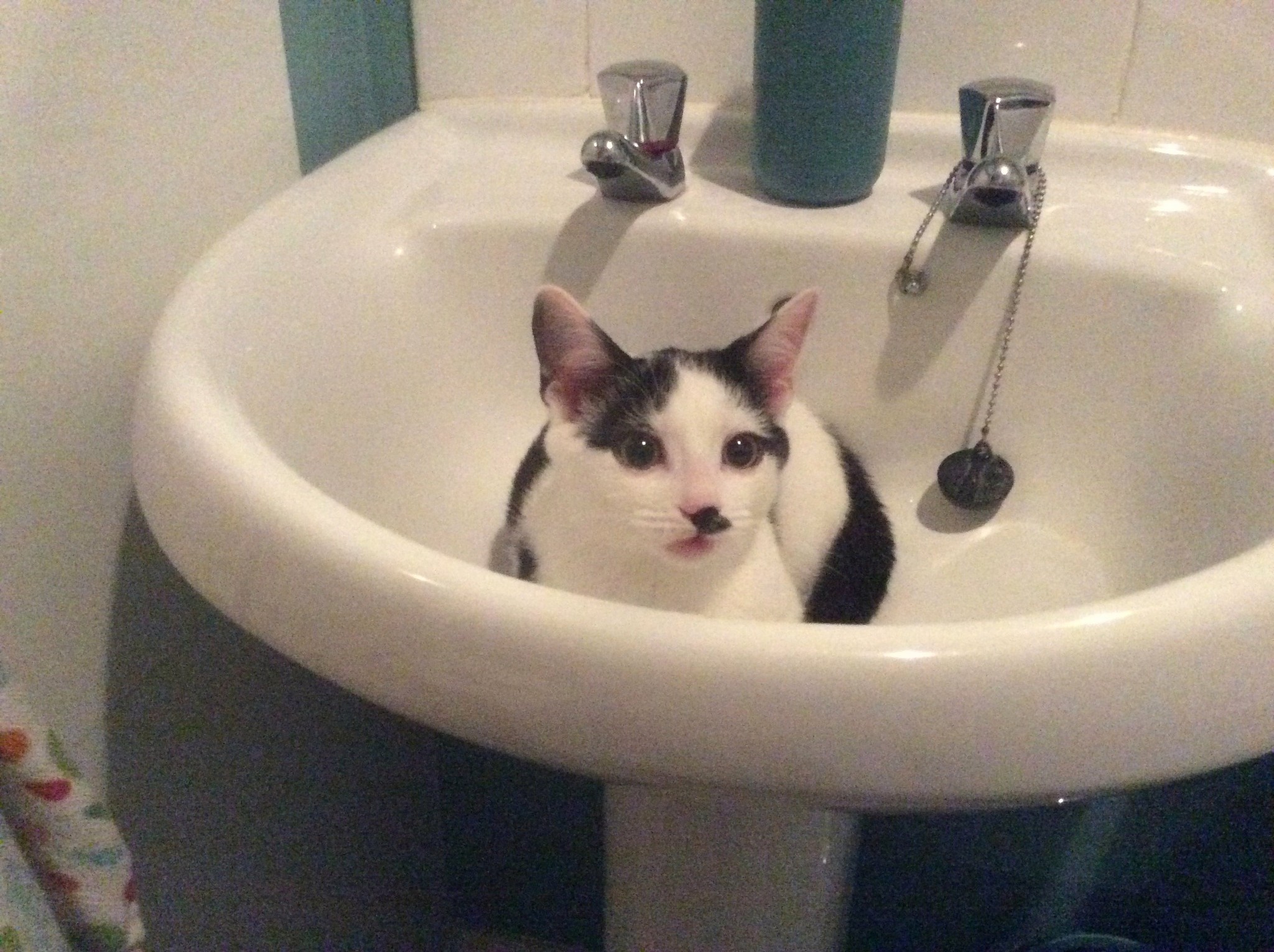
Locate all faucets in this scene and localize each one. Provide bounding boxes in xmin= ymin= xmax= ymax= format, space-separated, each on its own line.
xmin=938 ymin=76 xmax=1061 ymax=235
xmin=577 ymin=60 xmax=691 ymax=200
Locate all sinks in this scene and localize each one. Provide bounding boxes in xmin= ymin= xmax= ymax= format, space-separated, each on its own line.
xmin=131 ymin=96 xmax=1274 ymax=812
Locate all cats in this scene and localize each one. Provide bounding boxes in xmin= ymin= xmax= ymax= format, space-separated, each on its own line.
xmin=490 ymin=286 xmax=896 ymax=626
